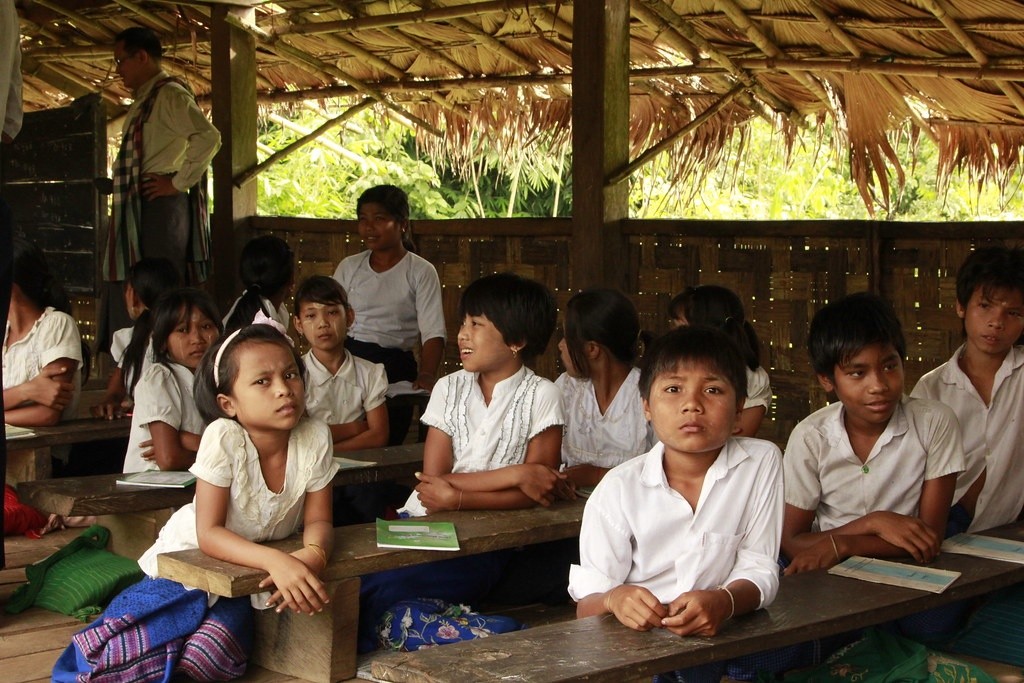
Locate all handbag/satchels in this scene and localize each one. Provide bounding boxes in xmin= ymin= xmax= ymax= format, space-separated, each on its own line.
xmin=3 ymin=523 xmax=144 ymax=622
xmin=783 ymin=627 xmax=992 ymax=683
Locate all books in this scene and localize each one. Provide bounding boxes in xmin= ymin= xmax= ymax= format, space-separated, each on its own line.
xmin=5 ymin=423 xmax=37 ymax=440
xmin=938 ymin=532 xmax=1024 ymax=564
xmin=826 ymin=555 xmax=962 ymax=595
xmin=376 ymin=518 xmax=461 ymax=552
xmin=116 ymin=469 xmax=197 ymax=488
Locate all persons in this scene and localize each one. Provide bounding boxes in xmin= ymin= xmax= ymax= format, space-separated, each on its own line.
xmin=555 ymin=287 xmax=660 ymax=492
xmin=137 ymin=324 xmax=341 ymax=616
xmin=358 ymin=272 xmax=578 ymax=652
xmin=1 ymin=236 xmax=85 ymax=491
xmin=93 ymin=27 xmax=223 ymax=301
xmin=292 ymin=275 xmax=389 ymax=452
xmin=782 ymin=291 xmax=976 ymax=667
xmin=331 ymin=185 xmax=448 ymax=446
xmin=667 ymin=286 xmax=773 ymax=438
xmin=909 ymin=239 xmax=1024 ymax=668
xmin=120 ymin=287 xmax=225 ymax=474
xmin=219 ymin=235 xmax=296 ymax=349
xmin=88 ymin=255 xmax=183 ymax=421
xmin=566 ymin=324 xmax=801 ymax=683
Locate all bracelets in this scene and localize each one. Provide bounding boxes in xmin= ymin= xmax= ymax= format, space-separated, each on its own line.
xmin=457 ymin=489 xmax=464 ymax=511
xmin=712 ymin=585 xmax=734 ymax=620
xmin=307 ymin=544 xmax=326 ymax=569
xmin=830 ymin=534 xmax=841 ymax=562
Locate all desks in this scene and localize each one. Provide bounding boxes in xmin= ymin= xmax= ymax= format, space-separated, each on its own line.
xmin=2 ymin=358 xmax=1024 ymax=683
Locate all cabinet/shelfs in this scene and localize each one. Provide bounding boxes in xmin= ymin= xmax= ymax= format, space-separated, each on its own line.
xmin=0 ymin=106 xmax=112 ymax=352
